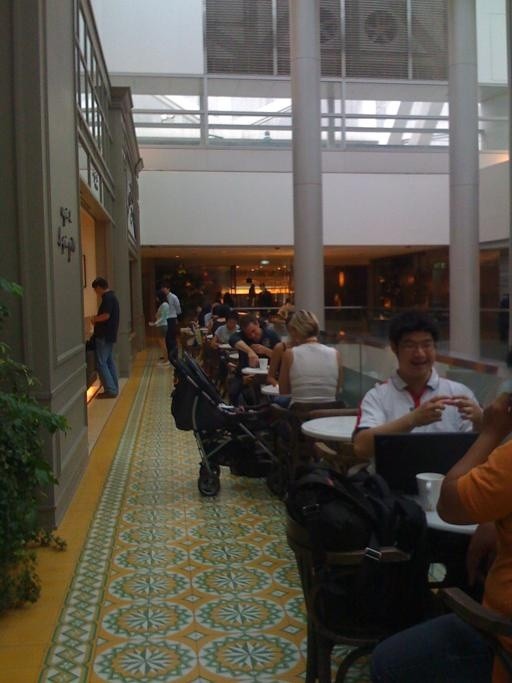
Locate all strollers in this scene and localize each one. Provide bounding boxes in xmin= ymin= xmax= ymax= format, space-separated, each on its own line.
xmin=169 ymin=348 xmax=286 ymax=498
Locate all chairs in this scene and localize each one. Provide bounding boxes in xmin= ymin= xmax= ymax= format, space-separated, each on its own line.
xmin=178 ymin=319 xmax=512 ymax=681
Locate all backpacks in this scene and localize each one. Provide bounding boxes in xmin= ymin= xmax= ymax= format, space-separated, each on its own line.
xmin=285 ymin=464 xmax=427 ymax=551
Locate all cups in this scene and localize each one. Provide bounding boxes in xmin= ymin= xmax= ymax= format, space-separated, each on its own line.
xmin=415 ymin=471 xmax=447 ymax=512
xmin=259 ymin=357 xmax=268 ymax=369
xmin=207 ymin=335 xmax=212 ymax=342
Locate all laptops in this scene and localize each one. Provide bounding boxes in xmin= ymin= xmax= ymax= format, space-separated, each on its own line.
xmin=371 ymin=432 xmax=479 ymax=493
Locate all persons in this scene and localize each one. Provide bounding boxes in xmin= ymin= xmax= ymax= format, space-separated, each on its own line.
xmin=498 ymin=295 xmax=509 ymax=340
xmin=367 ymin=293 xmax=511 ymax=682
xmin=351 ymin=308 xmax=484 ymax=476
xmin=191 ymin=283 xmax=344 ymax=416
xmin=90 ymin=276 xmax=120 ymax=398
xmin=148 ymin=283 xmax=180 ymax=365
